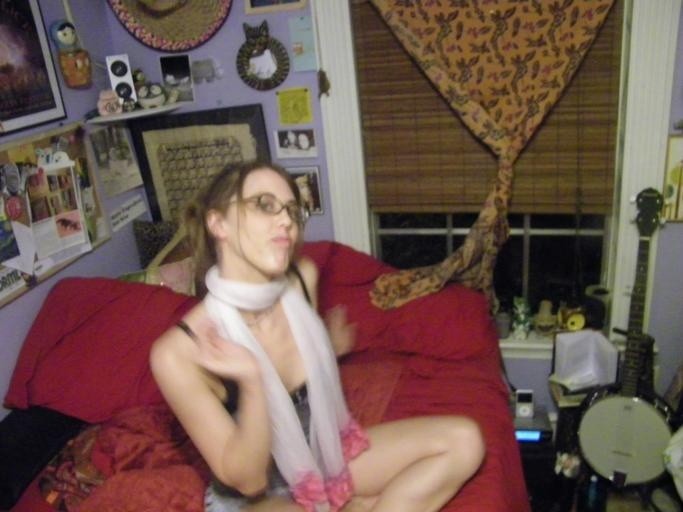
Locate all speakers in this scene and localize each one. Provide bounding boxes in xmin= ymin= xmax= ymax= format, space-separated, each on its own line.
xmin=106 ymin=53 xmax=138 ymax=106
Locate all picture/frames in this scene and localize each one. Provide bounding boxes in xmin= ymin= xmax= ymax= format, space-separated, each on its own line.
xmin=1 ymin=0 xmax=112 ymax=308
xmin=243 ymin=0 xmax=307 ymax=15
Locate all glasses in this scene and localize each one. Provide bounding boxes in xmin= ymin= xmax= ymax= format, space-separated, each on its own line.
xmin=220 ymin=192 xmax=311 ymax=223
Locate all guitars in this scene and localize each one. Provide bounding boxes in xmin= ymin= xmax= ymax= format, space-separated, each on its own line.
xmin=576 ymin=187 xmax=678 ymax=491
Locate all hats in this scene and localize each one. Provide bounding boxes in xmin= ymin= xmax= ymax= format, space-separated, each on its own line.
xmin=108 ymin=0 xmax=232 ymax=53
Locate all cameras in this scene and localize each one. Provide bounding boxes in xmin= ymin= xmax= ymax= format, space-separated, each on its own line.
xmin=515 ymin=389 xmax=536 ymax=419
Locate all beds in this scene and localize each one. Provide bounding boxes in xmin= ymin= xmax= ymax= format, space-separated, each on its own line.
xmin=1 ymin=268 xmax=529 ymax=509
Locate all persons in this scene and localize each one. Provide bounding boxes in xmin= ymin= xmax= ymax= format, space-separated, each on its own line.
xmin=284 ymin=132 xmax=298 ymax=150
xmin=295 ymin=134 xmax=314 ymax=153
xmin=146 ymin=160 xmax=488 ymax=511
